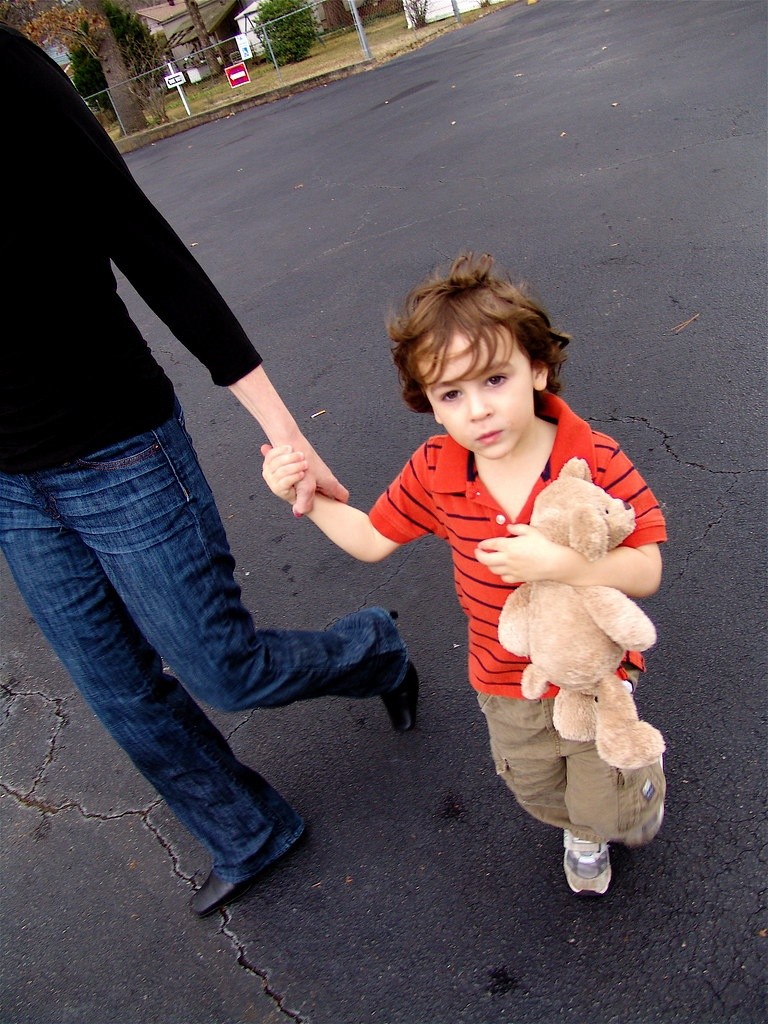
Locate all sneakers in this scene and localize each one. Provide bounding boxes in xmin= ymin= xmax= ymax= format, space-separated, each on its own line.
xmin=563 ymin=827 xmax=611 ymax=896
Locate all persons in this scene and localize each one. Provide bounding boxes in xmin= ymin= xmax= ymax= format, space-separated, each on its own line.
xmin=263 ymin=254 xmax=668 ymax=899
xmin=0 ymin=21 xmax=424 ymax=917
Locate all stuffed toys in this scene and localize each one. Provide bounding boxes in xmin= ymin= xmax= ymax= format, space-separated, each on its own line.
xmin=494 ymin=457 xmax=666 ymax=770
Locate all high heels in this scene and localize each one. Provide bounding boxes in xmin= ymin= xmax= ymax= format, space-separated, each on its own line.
xmin=188 ymin=872 xmax=242 ymax=918
xmin=378 ymin=609 xmax=419 ymax=731
